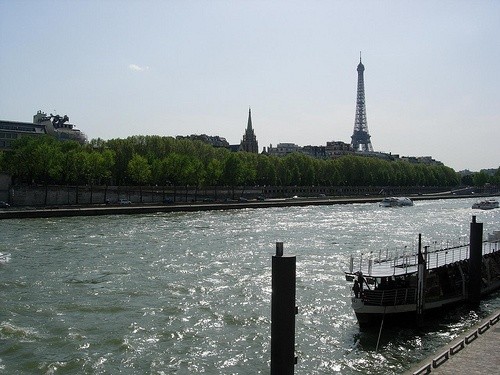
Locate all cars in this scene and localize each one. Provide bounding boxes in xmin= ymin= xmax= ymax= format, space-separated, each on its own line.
xmin=0 ymin=201 xmax=10 ymax=208
xmin=117 ymin=199 xmax=132 ymax=205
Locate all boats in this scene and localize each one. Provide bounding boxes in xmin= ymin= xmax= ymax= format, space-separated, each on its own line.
xmin=379 ymin=196 xmax=414 ymax=208
xmin=343 ymin=230 xmax=500 ymax=334
xmin=471 ymin=198 xmax=500 ymax=210
